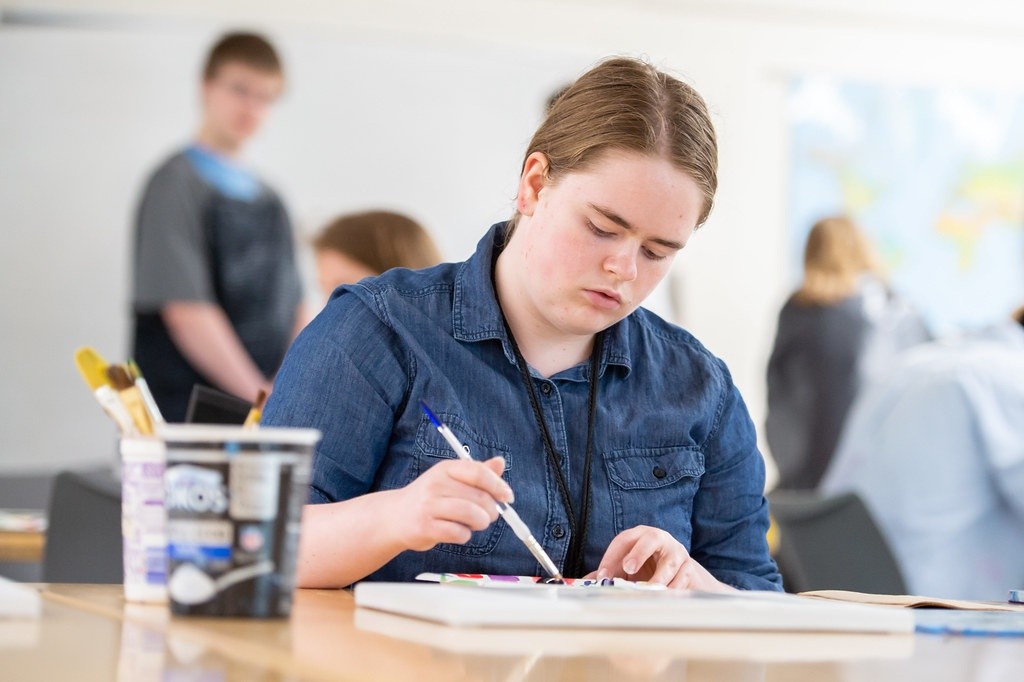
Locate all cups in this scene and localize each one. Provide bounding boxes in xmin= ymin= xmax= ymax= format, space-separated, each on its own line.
xmin=159 ymin=422 xmax=324 ymax=620
xmin=119 ymin=435 xmax=165 ymax=604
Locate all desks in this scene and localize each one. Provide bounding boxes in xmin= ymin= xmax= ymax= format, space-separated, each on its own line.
xmin=0 ymin=584 xmax=1024 ymax=682
xmin=0 ymin=510 xmax=49 ymax=585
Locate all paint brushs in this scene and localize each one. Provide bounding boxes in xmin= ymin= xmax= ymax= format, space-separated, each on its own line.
xmin=418 ymin=398 xmax=565 ymax=577
xmin=70 ymin=349 xmax=269 ymax=455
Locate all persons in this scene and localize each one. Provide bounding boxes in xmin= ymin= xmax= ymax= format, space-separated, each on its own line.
xmin=766 ymin=214 xmax=889 ymax=490
xmin=822 ymin=306 xmax=1024 ymax=602
xmin=315 ymin=212 xmax=445 ymax=310
xmin=260 ymin=59 xmax=786 ymax=591
xmin=131 ymin=31 xmax=309 ymax=426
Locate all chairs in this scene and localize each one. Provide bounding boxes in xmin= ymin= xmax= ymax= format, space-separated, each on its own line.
xmin=42 ymin=468 xmax=123 ymax=583
xmin=766 ymin=487 xmax=912 ymax=596
